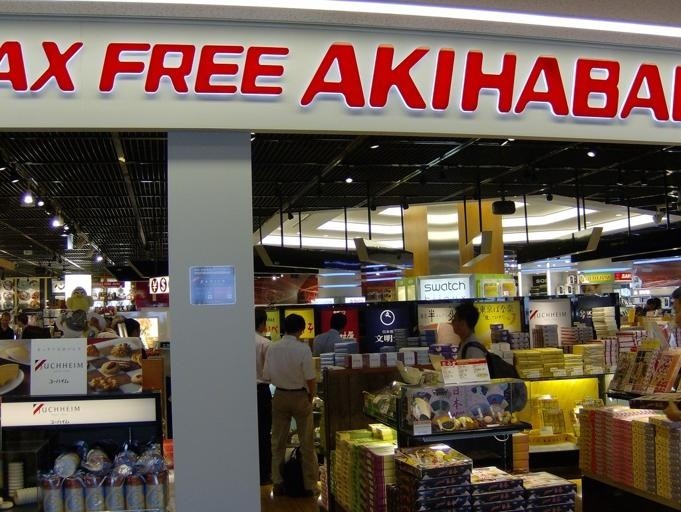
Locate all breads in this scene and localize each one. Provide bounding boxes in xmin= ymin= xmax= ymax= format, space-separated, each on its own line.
xmin=88 ymin=342 xmax=143 ymax=392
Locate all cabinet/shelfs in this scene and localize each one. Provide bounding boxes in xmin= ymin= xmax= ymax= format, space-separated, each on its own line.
xmin=1 ymin=335 xmax=166 ymax=511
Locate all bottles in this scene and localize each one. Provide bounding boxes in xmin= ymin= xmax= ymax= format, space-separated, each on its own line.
xmin=0 ymin=441 xmax=168 ymax=512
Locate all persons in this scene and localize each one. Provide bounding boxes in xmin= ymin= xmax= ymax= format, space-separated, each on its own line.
xmin=450 ymin=302 xmax=487 ymax=362
xmin=664 ymin=287 xmax=680 ymax=423
xmin=261 ymin=314 xmax=318 ymax=495
xmin=0 ymin=312 xmax=13 ymax=340
xmin=255 ymin=306 xmax=273 ymax=487
xmin=13 ymin=314 xmax=28 ymax=340
xmin=646 ymin=296 xmax=661 ymax=311
xmin=123 ymin=318 xmax=147 ymax=359
xmin=311 ymin=312 xmax=347 ymax=356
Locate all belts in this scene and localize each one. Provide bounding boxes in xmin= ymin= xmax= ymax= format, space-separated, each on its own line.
xmin=276 ymin=387 xmax=305 ymax=393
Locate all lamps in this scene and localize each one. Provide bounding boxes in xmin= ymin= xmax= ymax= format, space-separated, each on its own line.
xmin=587 ymin=143 xmax=599 ymax=158
xmin=496 ymin=137 xmax=515 ymax=147
xmin=59 ymin=231 xmax=70 ymax=237
xmin=249 ymin=134 xmax=257 ymax=142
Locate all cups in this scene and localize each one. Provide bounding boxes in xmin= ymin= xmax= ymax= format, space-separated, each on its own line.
xmin=558 ymin=273 xmax=577 ymax=295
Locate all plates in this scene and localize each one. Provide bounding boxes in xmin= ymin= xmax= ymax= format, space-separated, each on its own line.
xmin=0 ymin=369 xmax=24 ymax=394
xmin=3 ymin=279 xmax=40 ymax=310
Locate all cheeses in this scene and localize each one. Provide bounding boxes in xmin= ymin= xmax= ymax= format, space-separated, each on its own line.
xmin=0 ymin=363 xmax=18 ymax=386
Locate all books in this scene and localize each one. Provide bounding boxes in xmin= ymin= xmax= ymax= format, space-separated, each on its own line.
xmin=575 ymin=403 xmax=680 ymax=500
xmin=514 ymin=329 xmax=647 ymax=379
xmin=320 ymin=341 xmax=352 ymax=379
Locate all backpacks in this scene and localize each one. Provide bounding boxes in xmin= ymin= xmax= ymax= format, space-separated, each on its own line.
xmin=462 ymin=342 xmax=527 ymax=412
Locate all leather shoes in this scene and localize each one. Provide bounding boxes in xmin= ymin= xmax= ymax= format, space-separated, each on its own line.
xmin=260 ymin=479 xmax=321 ymax=497
